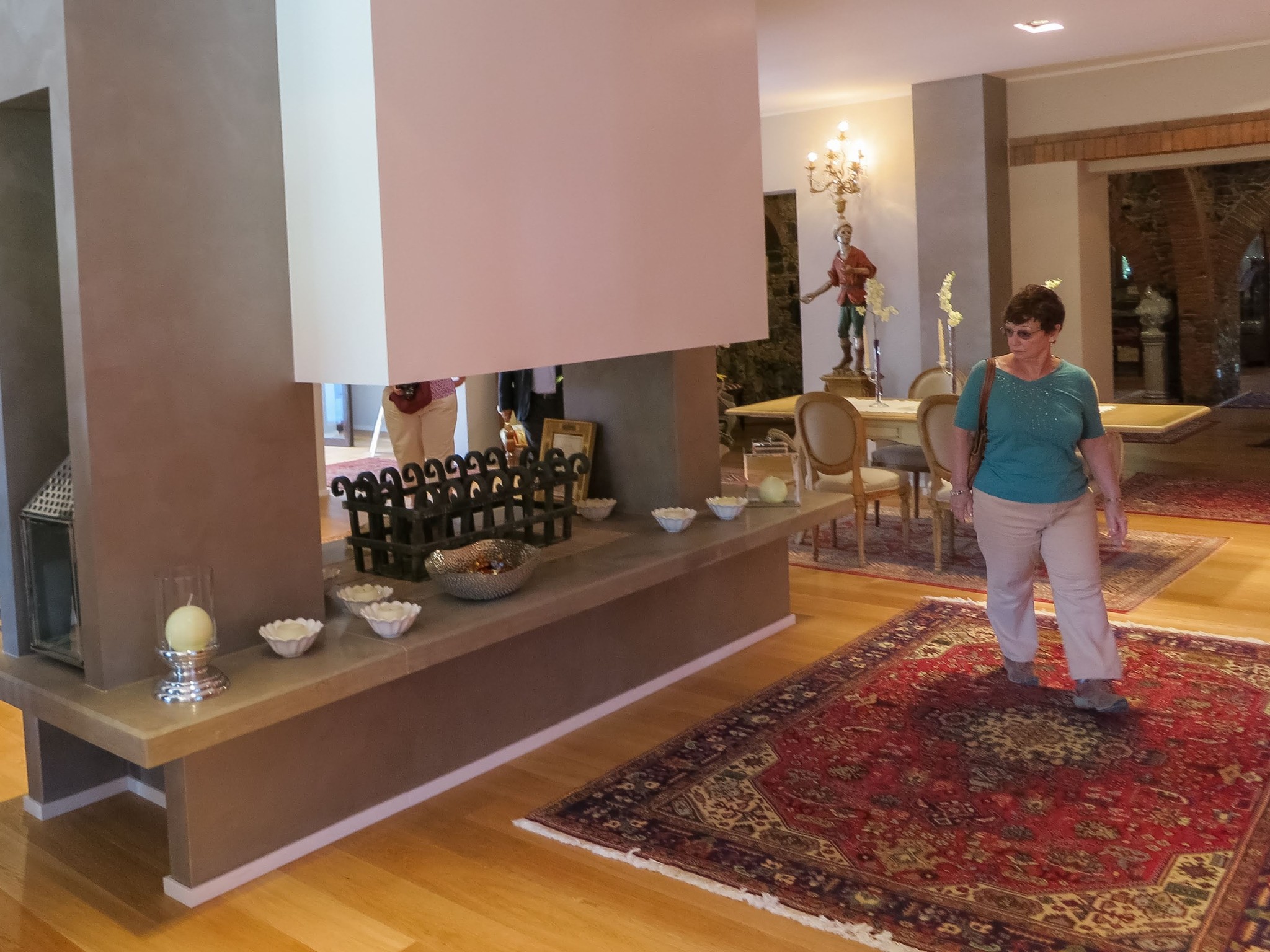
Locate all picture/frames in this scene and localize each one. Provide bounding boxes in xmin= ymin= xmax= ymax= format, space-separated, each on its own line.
xmin=533 ymin=419 xmax=598 ymax=508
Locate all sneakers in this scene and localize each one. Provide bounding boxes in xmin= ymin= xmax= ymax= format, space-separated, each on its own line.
xmin=1004 ymin=657 xmax=1038 ymax=685
xmin=1074 ymin=679 xmax=1127 ymax=712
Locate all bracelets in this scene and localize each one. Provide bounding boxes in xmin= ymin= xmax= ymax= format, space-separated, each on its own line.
xmin=950 ymin=487 xmax=969 ymax=496
xmin=1103 ymin=496 xmax=1124 ymax=503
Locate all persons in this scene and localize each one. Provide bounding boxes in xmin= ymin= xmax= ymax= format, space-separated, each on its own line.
xmin=798 ymin=220 xmax=877 ymax=374
xmin=382 ymin=376 xmax=466 ymax=509
xmin=949 ymin=284 xmax=1129 ymax=713
xmin=498 ymin=365 xmax=565 ymax=462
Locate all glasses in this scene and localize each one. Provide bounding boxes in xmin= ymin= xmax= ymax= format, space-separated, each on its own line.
xmin=999 ymin=326 xmax=1043 ymax=339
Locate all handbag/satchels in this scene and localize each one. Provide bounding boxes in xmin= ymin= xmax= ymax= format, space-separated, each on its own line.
xmin=389 ymin=382 xmax=431 ymax=414
xmin=967 ymin=426 xmax=987 ymax=491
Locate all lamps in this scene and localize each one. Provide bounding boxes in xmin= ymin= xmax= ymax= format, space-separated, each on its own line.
xmin=805 ymin=120 xmax=866 ymax=218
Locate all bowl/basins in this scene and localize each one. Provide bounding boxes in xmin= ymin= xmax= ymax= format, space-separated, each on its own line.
xmin=651 ymin=507 xmax=697 ymax=532
xmin=705 ymin=496 xmax=749 ymax=521
xmin=257 ymin=617 xmax=323 ymax=658
xmin=336 ymin=583 xmax=397 ymax=618
xmin=574 ymin=497 xmax=618 ymax=521
xmin=424 ymin=538 xmax=543 ymax=599
xmin=359 ymin=600 xmax=422 ymax=638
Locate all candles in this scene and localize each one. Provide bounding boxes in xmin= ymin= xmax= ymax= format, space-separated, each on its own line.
xmin=936 ymin=318 xmax=946 ymax=362
xmin=863 ymin=326 xmax=870 ymax=371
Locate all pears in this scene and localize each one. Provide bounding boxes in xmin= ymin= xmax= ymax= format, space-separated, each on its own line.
xmin=759 ymin=475 xmax=788 ymax=504
xmin=165 ymin=594 xmax=213 ymax=652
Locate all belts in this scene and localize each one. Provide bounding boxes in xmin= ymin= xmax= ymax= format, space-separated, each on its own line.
xmin=532 ymin=391 xmax=557 ymax=400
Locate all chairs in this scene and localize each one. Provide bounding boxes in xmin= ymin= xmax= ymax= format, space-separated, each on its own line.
xmin=1113 ymin=316 xmax=1143 ymax=377
xmin=794 ymin=366 xmax=977 ymax=570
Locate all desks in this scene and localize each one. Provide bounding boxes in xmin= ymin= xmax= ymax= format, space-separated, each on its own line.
xmin=720 ymin=394 xmax=1215 ymax=545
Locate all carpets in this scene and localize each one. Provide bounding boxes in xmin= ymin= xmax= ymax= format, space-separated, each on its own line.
xmin=782 ymin=503 xmax=1229 ymax=614
xmin=1120 ymin=420 xmax=1219 ymax=444
xmin=1218 ymin=390 xmax=1270 ymax=409
xmin=512 ymin=599 xmax=1270 ymax=952
xmin=1096 ymin=472 xmax=1270 ymax=524
